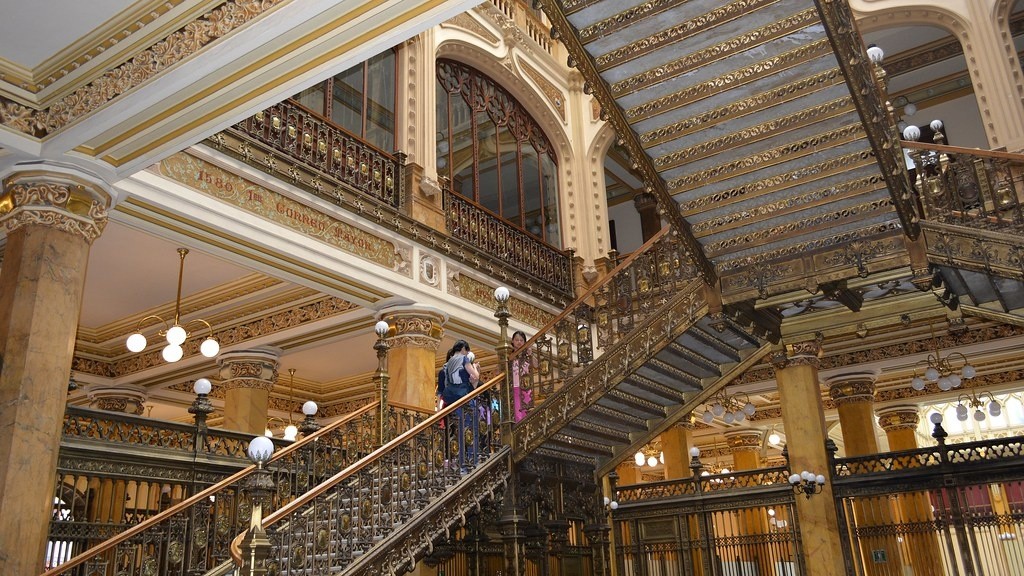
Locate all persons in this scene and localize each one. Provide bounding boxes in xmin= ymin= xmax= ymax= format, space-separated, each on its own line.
xmin=436 ymin=340 xmax=481 ymax=467
xmin=494 ymin=332 xmax=538 ymax=424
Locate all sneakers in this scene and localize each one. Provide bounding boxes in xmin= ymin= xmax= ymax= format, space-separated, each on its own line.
xmin=443 ymin=459 xmax=459 ymax=470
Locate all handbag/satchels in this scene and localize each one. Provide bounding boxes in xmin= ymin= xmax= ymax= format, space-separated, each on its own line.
xmin=443 ymin=385 xmax=467 ymax=404
xmin=520 ymin=374 xmax=532 ymax=391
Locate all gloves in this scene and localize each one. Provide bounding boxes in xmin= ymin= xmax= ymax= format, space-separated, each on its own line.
xmin=479 ymin=407 xmax=485 ymax=419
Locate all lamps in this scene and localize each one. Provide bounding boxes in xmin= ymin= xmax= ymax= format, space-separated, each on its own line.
xmin=494 ymin=285 xmax=512 ymax=318
xmin=188 ymin=378 xmax=215 ymax=414
xmin=930 ymin=120 xmax=945 ymax=141
xmin=866 ymin=47 xmax=888 ymax=80
xmin=768 ymin=508 xmax=786 ymax=532
xmin=911 ymin=317 xmax=975 ymax=391
xmin=768 ymin=424 xmax=781 ymax=445
xmin=372 ymin=320 xmax=390 ymax=350
xmin=634 ymin=441 xmax=664 ymax=468
xmin=701 ymin=436 xmax=734 ymax=484
xmin=903 ymin=125 xmax=923 ymax=157
xmin=265 ymin=368 xmax=304 ymax=441
xmin=126 ymin=246 xmax=220 ymax=362
xmin=930 ymin=412 xmax=948 ymax=437
xmin=689 ymin=447 xmax=703 ymax=468
xmin=604 ymin=496 xmax=619 ymax=520
xmin=787 ymin=471 xmax=825 ymax=500
xmin=243 ymin=435 xmax=277 ymax=492
xmin=298 ymin=400 xmax=320 ymax=431
xmin=956 ymin=378 xmax=1001 ymax=421
xmin=702 ymin=386 xmax=756 ymax=424
xmin=890 ymin=96 xmax=916 ymax=134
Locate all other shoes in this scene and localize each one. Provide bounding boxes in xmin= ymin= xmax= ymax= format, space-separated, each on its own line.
xmin=460 ymin=463 xmax=478 ymax=475
